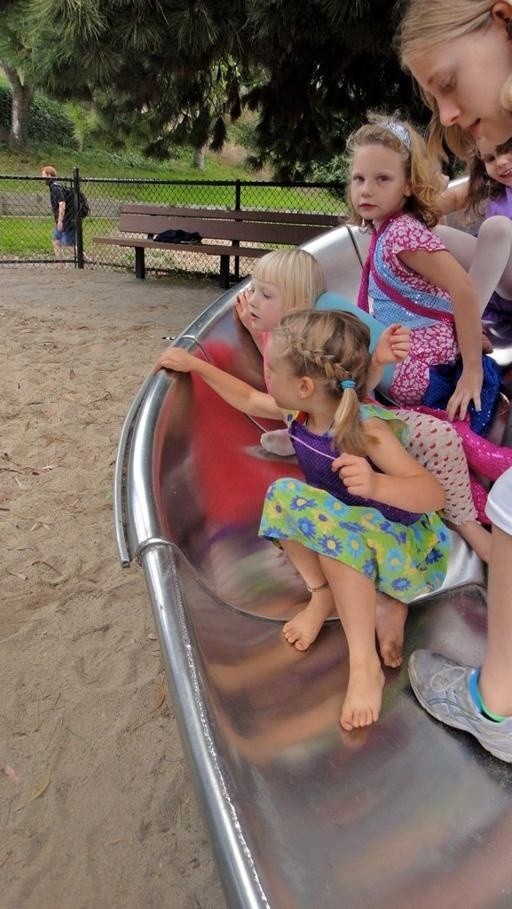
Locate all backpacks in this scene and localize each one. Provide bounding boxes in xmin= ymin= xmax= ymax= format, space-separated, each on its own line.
xmin=50 ymin=181 xmax=88 ymax=219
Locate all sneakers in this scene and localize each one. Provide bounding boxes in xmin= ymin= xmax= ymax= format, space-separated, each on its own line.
xmin=407 ymin=648 xmax=511 ymax=763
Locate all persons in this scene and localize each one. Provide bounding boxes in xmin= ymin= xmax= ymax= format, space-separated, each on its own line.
xmin=404 ymin=458 xmax=512 ymax=768
xmin=256 ymin=310 xmax=444 ymax=733
xmin=312 ymin=105 xmax=500 ymax=439
xmin=428 ymin=133 xmax=512 ymax=319
xmin=233 ymin=247 xmax=492 ymax=564
xmin=41 ymin=166 xmax=76 ymax=270
xmin=396 ymin=1 xmax=512 ymax=146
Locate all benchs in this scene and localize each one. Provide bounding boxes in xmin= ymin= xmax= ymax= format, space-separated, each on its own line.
xmin=92 ymin=203 xmax=350 ymax=291
